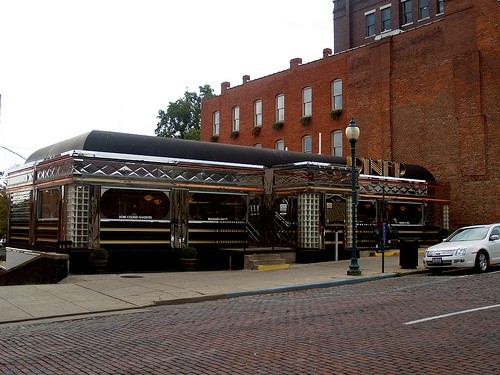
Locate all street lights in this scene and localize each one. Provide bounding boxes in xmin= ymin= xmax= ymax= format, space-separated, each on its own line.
xmin=345 ymin=116 xmax=363 ymax=276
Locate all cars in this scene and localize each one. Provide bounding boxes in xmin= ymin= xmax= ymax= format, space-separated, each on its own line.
xmin=422 ymin=223 xmax=499 ymax=273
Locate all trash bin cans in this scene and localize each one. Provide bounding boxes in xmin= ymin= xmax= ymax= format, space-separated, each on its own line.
xmin=56 ymin=240 xmax=72 ymax=271
xmin=398 ymin=236 xmax=420 ymax=270
